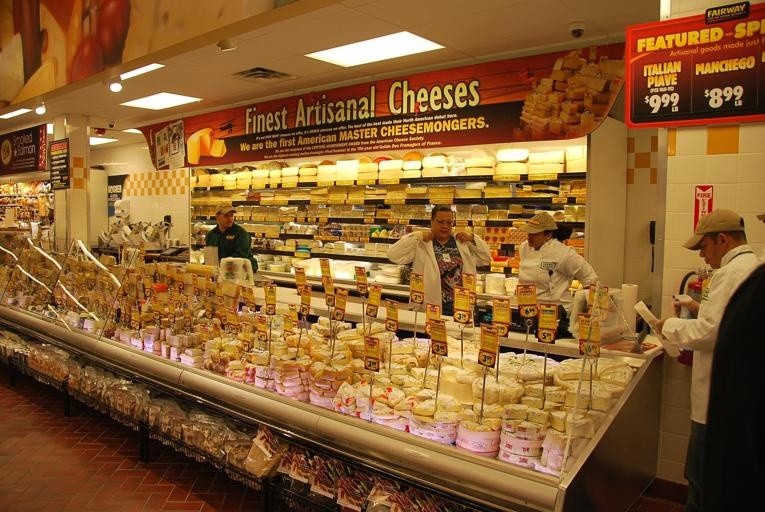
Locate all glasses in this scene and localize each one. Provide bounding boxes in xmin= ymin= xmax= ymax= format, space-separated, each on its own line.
xmin=434 ymin=217 xmax=454 ymax=226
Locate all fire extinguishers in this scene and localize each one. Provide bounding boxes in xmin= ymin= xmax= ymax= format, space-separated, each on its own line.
xmin=676 ymin=267 xmax=713 ymax=366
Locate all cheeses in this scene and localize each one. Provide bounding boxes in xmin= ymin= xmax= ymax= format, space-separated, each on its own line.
xmin=337 ymin=321 xmax=633 ymax=437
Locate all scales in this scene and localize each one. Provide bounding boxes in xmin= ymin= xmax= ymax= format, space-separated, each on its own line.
xmin=474 ymin=299 xmax=567 ymax=334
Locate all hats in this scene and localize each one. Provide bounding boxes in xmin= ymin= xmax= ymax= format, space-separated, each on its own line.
xmin=215 ymin=202 xmax=237 ymax=215
xmin=681 ymin=208 xmax=746 ymax=251
xmin=519 ymin=212 xmax=559 ymax=234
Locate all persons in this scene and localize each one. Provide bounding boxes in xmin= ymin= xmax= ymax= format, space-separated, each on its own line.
xmin=514 ymin=210 xmax=600 ymax=301
xmin=386 ymin=205 xmax=491 ymax=310
xmin=201 ymin=200 xmax=249 ymax=272
xmin=646 ymin=209 xmax=763 ymax=511
xmin=705 ymin=260 xmax=764 ymax=508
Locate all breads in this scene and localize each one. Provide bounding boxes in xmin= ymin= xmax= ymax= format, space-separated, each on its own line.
xmin=203 ymin=317 xmax=353 ymax=397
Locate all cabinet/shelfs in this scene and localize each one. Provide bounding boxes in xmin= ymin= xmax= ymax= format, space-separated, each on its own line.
xmin=192 ymin=115 xmax=626 ymax=316
xmin=0 ymin=170 xmax=66 ymax=251
